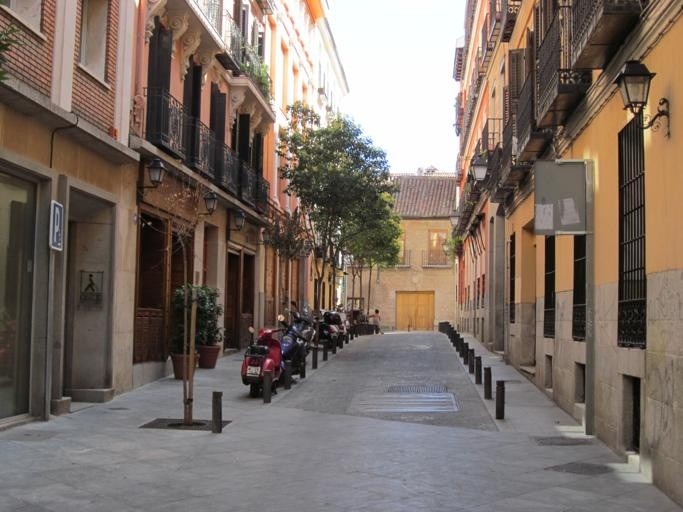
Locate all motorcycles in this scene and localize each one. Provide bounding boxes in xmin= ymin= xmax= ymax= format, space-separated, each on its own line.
xmin=240 ymin=300 xmax=361 ymax=398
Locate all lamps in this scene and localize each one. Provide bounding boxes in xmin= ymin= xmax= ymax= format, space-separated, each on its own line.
xmin=198 ymin=193 xmax=249 ymax=231
xmin=614 ymin=57 xmax=672 ymax=137
xmin=137 ymin=156 xmax=167 ymax=202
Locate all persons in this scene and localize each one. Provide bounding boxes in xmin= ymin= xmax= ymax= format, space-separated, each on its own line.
xmin=369 ymin=309 xmax=384 ymax=334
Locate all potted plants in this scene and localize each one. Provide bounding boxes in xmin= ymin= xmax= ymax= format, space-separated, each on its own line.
xmin=171 ymin=286 xmax=224 ymax=377
